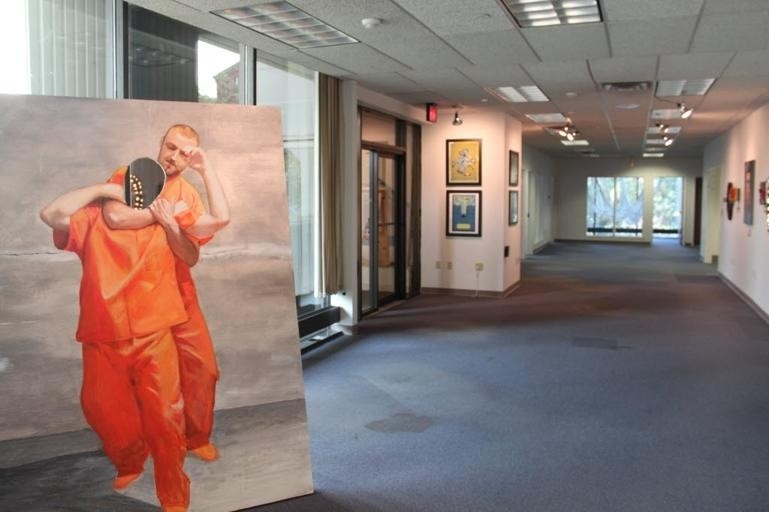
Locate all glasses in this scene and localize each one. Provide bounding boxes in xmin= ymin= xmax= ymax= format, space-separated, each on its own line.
xmin=452 ymin=103 xmax=465 ymax=127
xmin=654 ymin=96 xmax=694 ymax=146
xmin=559 ymin=124 xmax=579 ymax=142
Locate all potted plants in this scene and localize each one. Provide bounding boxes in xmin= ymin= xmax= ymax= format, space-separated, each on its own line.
xmin=445 ymin=138 xmax=483 ymax=238
xmin=508 ymin=148 xmax=519 ymax=226
